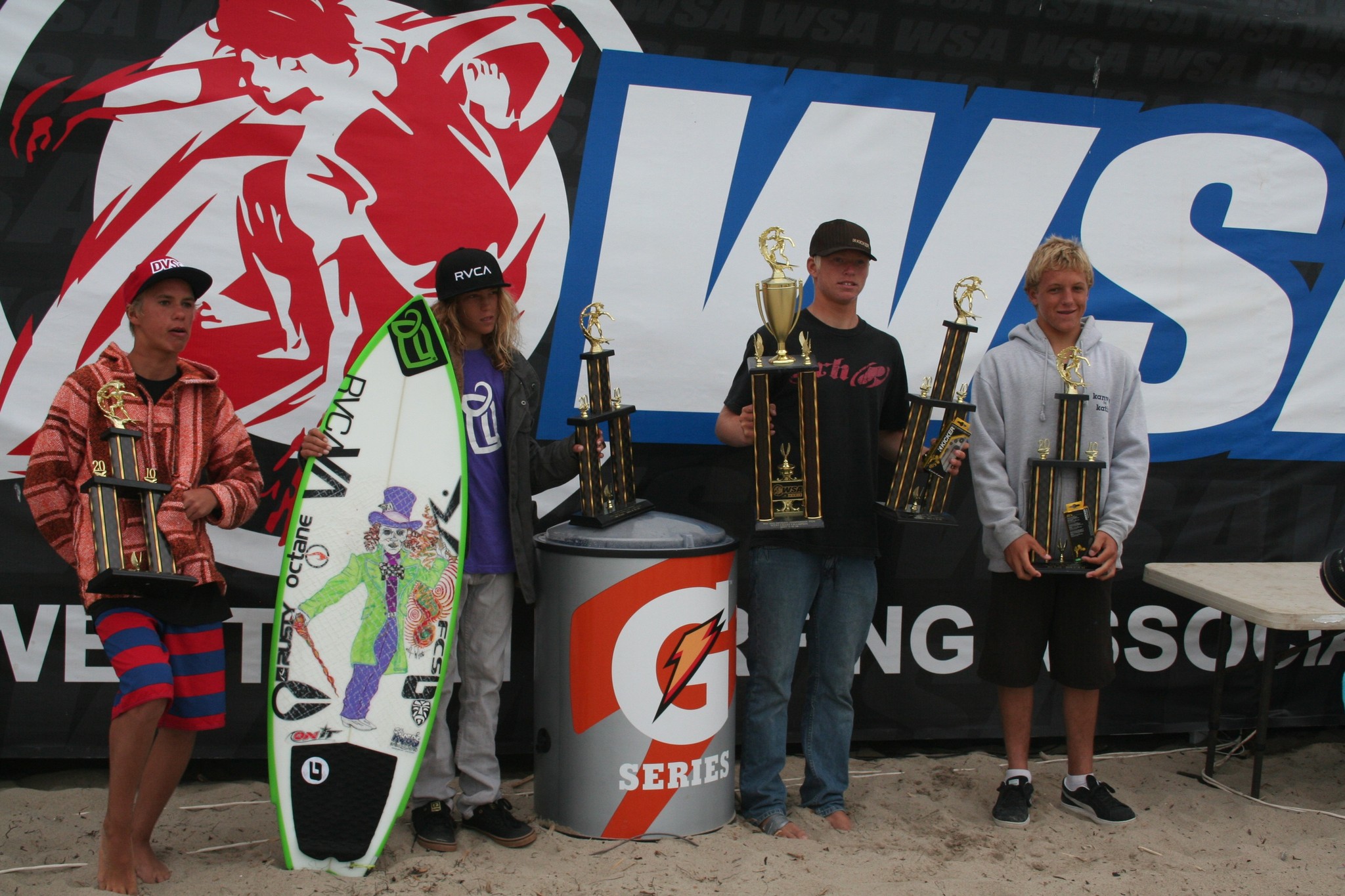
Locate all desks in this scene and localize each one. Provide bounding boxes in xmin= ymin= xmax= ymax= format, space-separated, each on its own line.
xmin=1143 ymin=561 xmax=1344 ymax=798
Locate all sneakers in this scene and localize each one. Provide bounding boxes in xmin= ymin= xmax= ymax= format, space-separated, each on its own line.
xmin=992 ymin=776 xmax=1034 ymax=828
xmin=461 ymin=798 xmax=538 ymax=848
xmin=410 ymin=800 xmax=457 ymax=852
xmin=1061 ymin=774 xmax=1137 ymax=827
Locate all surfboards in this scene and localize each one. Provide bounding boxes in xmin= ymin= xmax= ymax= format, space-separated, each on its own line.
xmin=267 ymin=292 xmax=469 ymax=878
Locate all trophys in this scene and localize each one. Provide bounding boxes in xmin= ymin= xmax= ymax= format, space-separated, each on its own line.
xmin=875 ymin=276 xmax=989 ymax=527
xmin=1028 ymin=347 xmax=1107 ymax=576
xmin=566 ymin=301 xmax=655 ymax=529
xmin=80 ymin=379 xmax=199 ymax=601
xmin=747 ymin=227 xmax=827 ymax=534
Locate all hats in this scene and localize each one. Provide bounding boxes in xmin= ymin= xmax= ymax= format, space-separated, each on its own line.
xmin=435 ymin=247 xmax=511 ymax=299
xmin=122 ymin=255 xmax=212 ymax=311
xmin=809 ymin=219 xmax=877 ymax=262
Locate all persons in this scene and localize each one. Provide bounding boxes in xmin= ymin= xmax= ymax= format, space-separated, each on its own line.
xmin=25 ymin=256 xmax=264 ymax=896
xmin=302 ymin=247 xmax=606 ymax=852
xmin=714 ymin=219 xmax=948 ymax=840
xmin=968 ymin=236 xmax=1150 ymax=829
